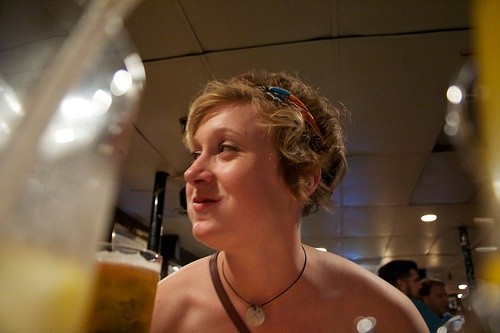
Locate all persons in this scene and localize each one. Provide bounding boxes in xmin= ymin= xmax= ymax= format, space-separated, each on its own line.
xmin=377 ymin=259 xmax=442 ymax=333
xmin=419 ymin=278 xmax=456 ymax=326
xmin=149 ymin=72 xmax=433 ymax=333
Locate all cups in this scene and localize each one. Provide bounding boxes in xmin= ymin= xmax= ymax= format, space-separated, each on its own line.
xmin=88 ymin=241 xmax=162 ymax=332
xmin=446 ymin=1 xmax=498 ymax=317
xmin=0 ymin=0 xmax=145 ymax=333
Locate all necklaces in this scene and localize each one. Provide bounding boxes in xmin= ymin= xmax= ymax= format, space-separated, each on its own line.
xmin=223 ymin=244 xmax=308 ymax=327
